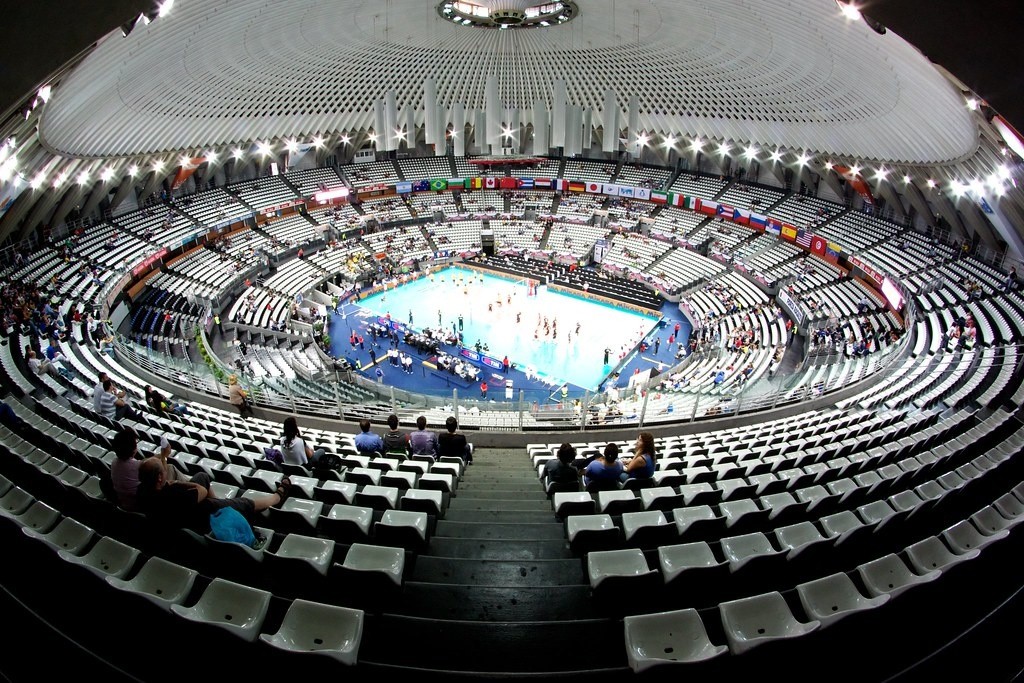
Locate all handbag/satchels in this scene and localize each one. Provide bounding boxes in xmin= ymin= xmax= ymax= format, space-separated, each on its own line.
xmin=210 ymin=506 xmax=256 ymax=547
xmin=264 ymin=448 xmax=285 ymax=464
xmin=243 ymin=396 xmax=254 ymax=414
xmin=58 ymin=367 xmax=75 ymax=381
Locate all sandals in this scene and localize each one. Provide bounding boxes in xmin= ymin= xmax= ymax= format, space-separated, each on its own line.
xmin=272 ymin=477 xmax=291 ymax=509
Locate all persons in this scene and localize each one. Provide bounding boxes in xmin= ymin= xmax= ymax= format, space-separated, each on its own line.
xmin=279 ymin=417 xmax=326 ymax=472
xmin=354 ymin=414 xmax=474 ymax=464
xmin=111 ymin=429 xmax=292 ymax=531
xmin=539 ymin=432 xmax=656 ymax=502
xmin=0 ymin=153 xmax=1024 ymax=421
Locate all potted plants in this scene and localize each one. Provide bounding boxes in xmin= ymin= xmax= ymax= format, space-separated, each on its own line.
xmin=322 ymin=336 xmax=330 ymax=348
xmin=327 ymin=313 xmax=331 ymax=324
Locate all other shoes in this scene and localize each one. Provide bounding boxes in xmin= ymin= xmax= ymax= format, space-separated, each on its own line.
xmin=137 ymin=411 xmax=143 ymax=421
xmin=132 ymin=409 xmax=137 ymax=414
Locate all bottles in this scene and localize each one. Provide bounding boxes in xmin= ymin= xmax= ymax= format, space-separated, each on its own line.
xmin=161 ymin=437 xmax=169 ymax=450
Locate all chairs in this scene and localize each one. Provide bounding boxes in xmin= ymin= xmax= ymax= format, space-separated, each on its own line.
xmin=0 ymin=154 xmax=1024 ymax=673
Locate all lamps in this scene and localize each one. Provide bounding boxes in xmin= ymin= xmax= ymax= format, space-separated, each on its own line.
xmin=8 ymin=134 xmax=17 ymax=144
xmin=1011 ymin=177 xmax=1018 ymax=188
xmin=859 ymin=11 xmax=886 ymax=36
xmin=997 ymin=140 xmax=1022 ymax=166
xmin=143 ymin=6 xmax=159 ymax=25
xmin=120 ymin=14 xmax=142 ymax=38
xmin=962 ymin=90 xmax=999 ymax=124
xmin=20 ymin=92 xmax=39 ymax=120
